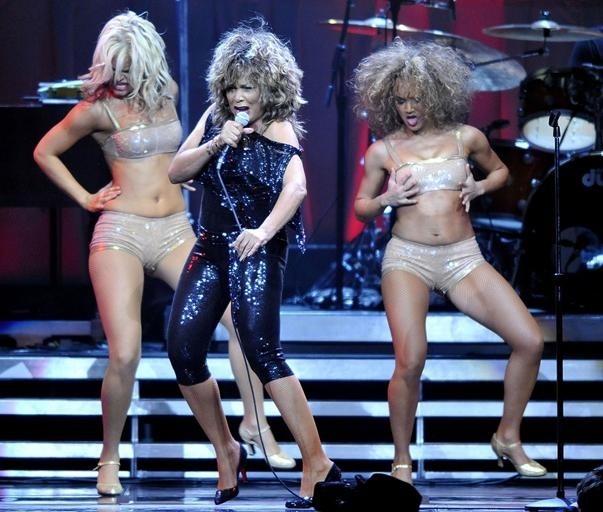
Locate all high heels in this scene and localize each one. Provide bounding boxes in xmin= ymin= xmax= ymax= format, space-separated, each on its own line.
xmin=214 ymin=442 xmax=248 ymax=505
xmin=491 ymin=433 xmax=547 ymax=477
xmin=239 ymin=422 xmax=297 ymax=469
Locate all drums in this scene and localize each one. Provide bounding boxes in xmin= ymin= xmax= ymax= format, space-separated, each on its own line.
xmin=471 ymin=139 xmax=544 ymax=232
xmin=520 ymin=150 xmax=602 ymax=314
xmin=517 ymin=64 xmax=602 ymax=154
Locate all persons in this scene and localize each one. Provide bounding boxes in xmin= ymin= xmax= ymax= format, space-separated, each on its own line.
xmin=348 ymin=38 xmax=549 ymax=489
xmin=165 ymin=19 xmax=341 ymax=510
xmin=33 ymin=9 xmax=298 ymax=498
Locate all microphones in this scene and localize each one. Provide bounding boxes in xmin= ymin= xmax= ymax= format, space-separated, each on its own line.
xmin=216 ymin=111 xmax=250 ymax=170
xmin=479 ymin=120 xmax=510 ymax=132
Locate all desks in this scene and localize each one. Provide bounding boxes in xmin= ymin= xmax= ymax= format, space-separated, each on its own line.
xmin=0 ymin=101 xmax=112 ymax=321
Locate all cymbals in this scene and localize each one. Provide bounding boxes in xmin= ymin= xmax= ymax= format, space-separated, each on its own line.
xmin=481 ymin=18 xmax=602 ymax=44
xmin=405 ymin=35 xmax=526 ymax=93
xmin=328 ymin=15 xmax=462 ymax=41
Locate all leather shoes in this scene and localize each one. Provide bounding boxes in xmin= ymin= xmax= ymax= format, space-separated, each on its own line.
xmin=93 ymin=460 xmax=124 ymax=496
xmin=391 ymin=463 xmax=414 ymax=486
xmin=285 ymin=463 xmax=342 ymax=509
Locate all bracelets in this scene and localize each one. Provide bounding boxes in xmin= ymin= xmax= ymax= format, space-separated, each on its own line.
xmin=201 ymin=134 xmax=223 ymax=159
